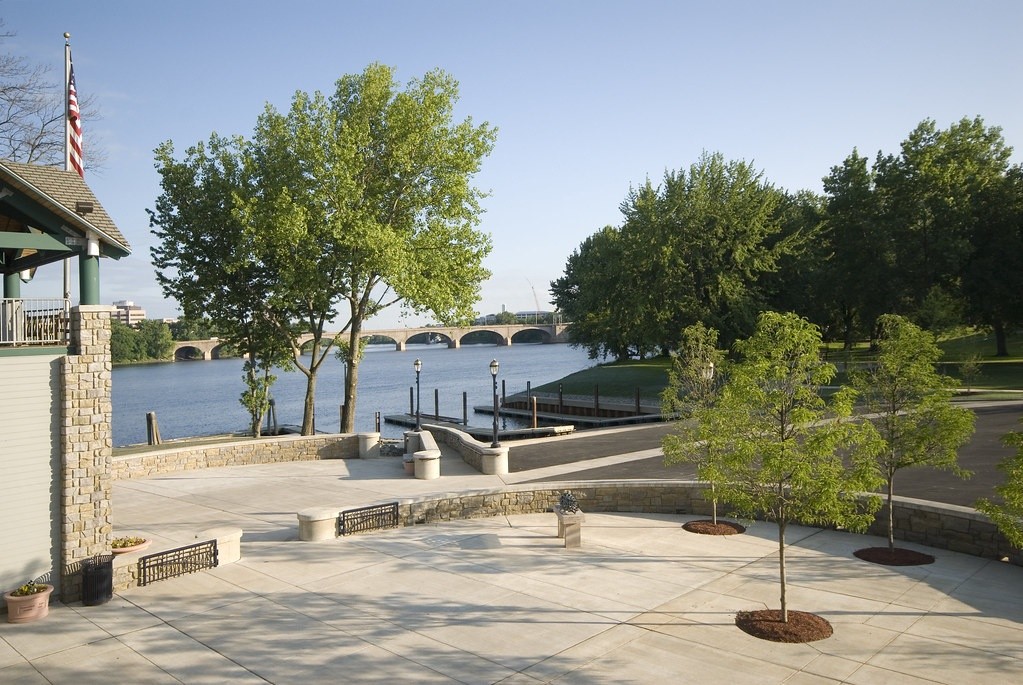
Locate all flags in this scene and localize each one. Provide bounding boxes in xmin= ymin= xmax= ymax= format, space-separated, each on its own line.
xmin=68 ymin=49 xmax=86 ymax=182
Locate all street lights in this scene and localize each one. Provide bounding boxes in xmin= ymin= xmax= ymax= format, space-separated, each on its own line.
xmin=489 ymin=359 xmax=501 ymax=448
xmin=702 ymin=357 xmax=714 ymax=407
xmin=414 ymin=358 xmax=423 ymax=432
